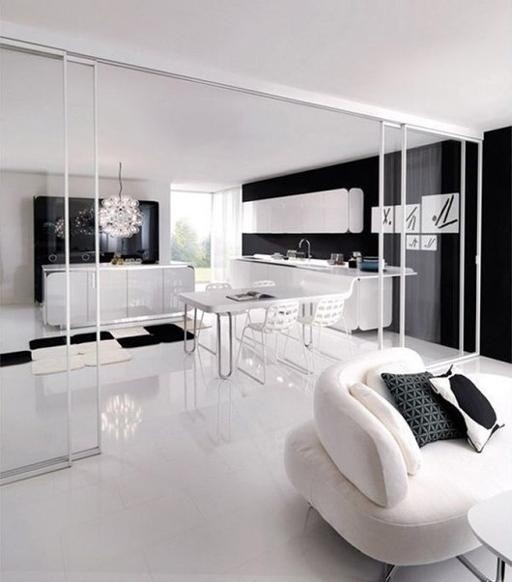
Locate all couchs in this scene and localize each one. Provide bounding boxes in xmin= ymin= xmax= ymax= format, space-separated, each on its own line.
xmin=284 ymin=347 xmax=510 ymax=581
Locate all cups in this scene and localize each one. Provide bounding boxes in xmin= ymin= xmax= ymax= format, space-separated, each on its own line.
xmin=331 ymin=253 xmax=343 ymax=264
xmin=287 ymin=250 xmax=306 ymax=261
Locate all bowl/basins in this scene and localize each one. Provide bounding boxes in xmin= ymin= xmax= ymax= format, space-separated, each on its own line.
xmin=271 ymin=253 xmax=288 ymax=260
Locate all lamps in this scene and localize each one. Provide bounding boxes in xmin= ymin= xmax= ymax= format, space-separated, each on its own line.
xmin=100 ymin=162 xmax=141 ymax=239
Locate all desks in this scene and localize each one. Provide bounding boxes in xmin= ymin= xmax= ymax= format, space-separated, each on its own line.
xmin=179 ymin=285 xmax=352 ymax=381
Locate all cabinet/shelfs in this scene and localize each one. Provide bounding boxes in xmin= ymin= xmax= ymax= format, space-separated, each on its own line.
xmin=42 ymin=268 xmax=195 ymax=328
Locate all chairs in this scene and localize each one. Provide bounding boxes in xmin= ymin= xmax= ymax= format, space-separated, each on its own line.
xmin=203 ymin=277 xmax=344 ymax=384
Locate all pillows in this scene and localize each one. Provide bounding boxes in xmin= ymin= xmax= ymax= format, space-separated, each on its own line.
xmin=382 ymin=363 xmax=505 ymax=452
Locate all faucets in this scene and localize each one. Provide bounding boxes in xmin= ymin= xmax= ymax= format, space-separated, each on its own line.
xmin=299 ymin=239 xmax=310 ymax=257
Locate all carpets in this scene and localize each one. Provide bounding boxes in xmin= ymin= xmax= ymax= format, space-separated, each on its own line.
xmin=0 ymin=316 xmax=195 ymax=378
